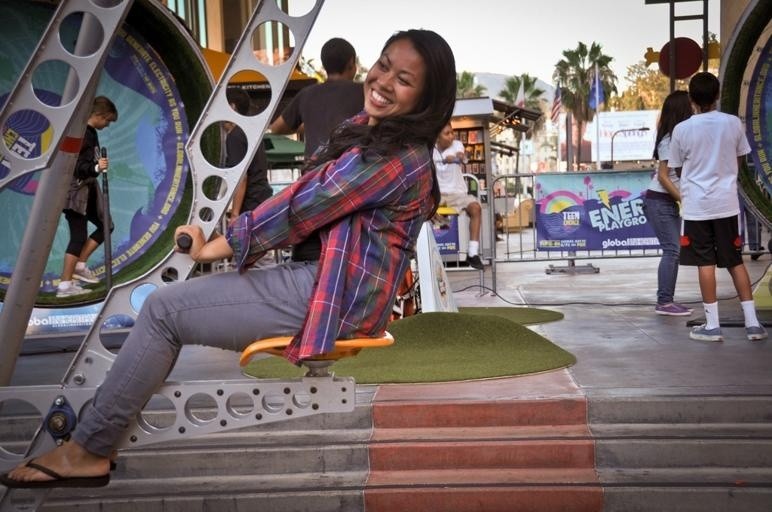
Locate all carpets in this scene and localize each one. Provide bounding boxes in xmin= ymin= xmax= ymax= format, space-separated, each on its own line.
xmin=457 ymin=306 xmax=565 ymax=327
xmin=241 ymin=312 xmax=578 ymax=384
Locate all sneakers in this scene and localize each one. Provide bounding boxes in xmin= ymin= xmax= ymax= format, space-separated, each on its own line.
xmin=466 ymin=254 xmax=483 ymax=269
xmin=744 ymin=323 xmax=768 ymax=341
xmin=689 ymin=323 xmax=724 ymax=342
xmin=55 ymin=283 xmax=91 ymax=298
xmin=655 ymin=301 xmax=694 ymax=316
xmin=71 ymin=265 xmax=100 ymax=284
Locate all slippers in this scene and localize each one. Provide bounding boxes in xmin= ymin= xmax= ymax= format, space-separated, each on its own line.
xmin=0 ymin=457 xmax=119 ymax=489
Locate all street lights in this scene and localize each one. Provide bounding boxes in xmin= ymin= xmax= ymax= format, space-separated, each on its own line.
xmin=610 ymin=126 xmax=650 ymax=169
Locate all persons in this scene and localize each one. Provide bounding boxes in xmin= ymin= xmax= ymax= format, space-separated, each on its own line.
xmin=222 ymin=87 xmax=275 ymax=271
xmin=667 ymin=70 xmax=768 ymax=343
xmin=56 ymin=94 xmax=119 ymax=299
xmin=643 ymin=89 xmax=697 ymax=318
xmin=433 ymin=119 xmax=488 ymax=273
xmin=0 ymin=26 xmax=458 ymax=491
xmin=271 ymin=36 xmax=367 ymax=168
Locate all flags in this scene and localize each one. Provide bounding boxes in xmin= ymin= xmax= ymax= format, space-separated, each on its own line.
xmin=514 ymin=78 xmax=526 ymax=110
xmin=551 ymin=80 xmax=562 ymax=125
xmin=589 ymin=72 xmax=605 ymax=109
